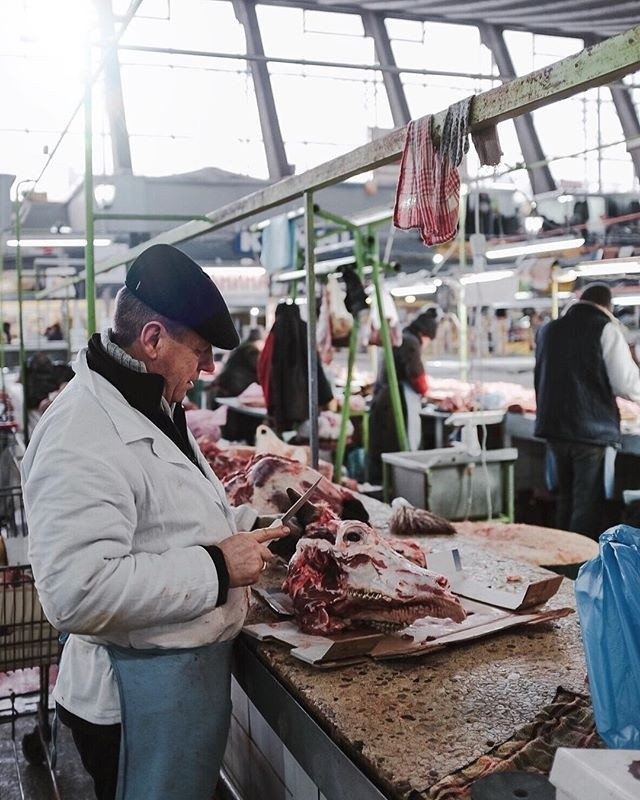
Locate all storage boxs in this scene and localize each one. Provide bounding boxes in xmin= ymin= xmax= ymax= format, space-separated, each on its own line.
xmin=549 ymin=748 xmax=640 ymax=800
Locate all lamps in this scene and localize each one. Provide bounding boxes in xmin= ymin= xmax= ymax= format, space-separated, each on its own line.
xmin=485 ymin=233 xmax=640 ymax=306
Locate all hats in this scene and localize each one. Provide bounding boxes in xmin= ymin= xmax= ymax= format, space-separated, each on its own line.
xmin=412 ymin=307 xmax=437 ymax=341
xmin=125 ymin=244 xmax=239 ymax=350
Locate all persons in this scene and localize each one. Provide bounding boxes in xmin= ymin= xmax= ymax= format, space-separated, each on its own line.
xmin=3 ymin=322 xmax=11 ymax=344
xmin=44 ymin=324 xmax=62 ymax=340
xmin=368 ymin=308 xmax=438 ymax=501
xmin=212 ymin=329 xmax=266 ymax=446
xmin=533 ymin=283 xmax=640 ymax=542
xmin=19 ymin=243 xmax=304 ymax=800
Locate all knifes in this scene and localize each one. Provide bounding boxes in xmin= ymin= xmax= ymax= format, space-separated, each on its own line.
xmin=261 ymin=474 xmax=323 ymax=547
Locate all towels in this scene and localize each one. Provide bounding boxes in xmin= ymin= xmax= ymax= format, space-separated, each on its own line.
xmin=391 ymin=91 xmax=504 ymax=247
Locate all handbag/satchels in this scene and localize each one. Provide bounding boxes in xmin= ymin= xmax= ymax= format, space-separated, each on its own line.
xmin=362 ymin=281 xmax=403 ymax=348
xmin=326 ymin=273 xmax=354 ymax=337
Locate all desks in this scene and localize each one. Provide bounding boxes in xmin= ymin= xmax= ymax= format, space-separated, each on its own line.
xmin=215 ymin=398 xmax=269 ymax=420
xmin=504 ymin=409 xmax=640 ymax=499
xmin=365 ymin=393 xmax=506 ymax=445
